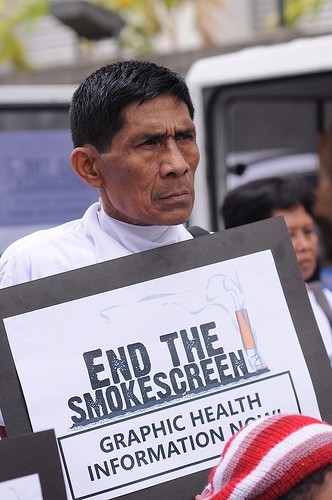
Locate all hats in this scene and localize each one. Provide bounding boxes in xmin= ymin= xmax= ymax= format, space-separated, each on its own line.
xmin=198 ymin=414 xmax=332 ymax=499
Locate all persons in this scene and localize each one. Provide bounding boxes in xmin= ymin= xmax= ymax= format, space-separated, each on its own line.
xmin=196 ymin=415 xmax=332 ymax=499
xmin=0 ymin=61 xmax=332 ymax=437
xmin=221 ymin=131 xmax=332 ymax=365
